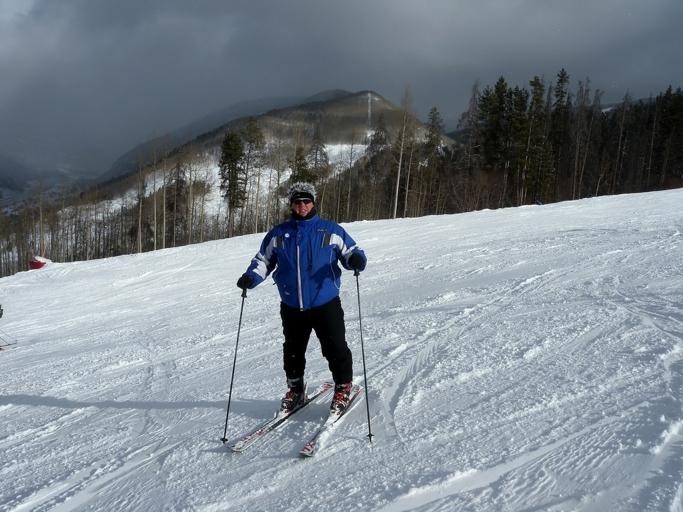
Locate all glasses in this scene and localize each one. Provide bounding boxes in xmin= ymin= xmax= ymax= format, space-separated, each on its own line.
xmin=294 ymin=199 xmax=311 ymax=204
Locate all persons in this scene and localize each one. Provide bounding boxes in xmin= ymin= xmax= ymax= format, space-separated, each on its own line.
xmin=235 ymin=181 xmax=368 ymax=416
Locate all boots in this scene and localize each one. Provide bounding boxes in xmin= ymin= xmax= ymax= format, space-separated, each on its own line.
xmin=330 ymin=382 xmax=352 ymax=412
xmin=281 ymin=388 xmax=307 ymax=409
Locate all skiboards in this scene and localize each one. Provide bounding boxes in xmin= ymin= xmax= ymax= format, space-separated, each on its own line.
xmin=228 ymin=381 xmax=363 ymax=456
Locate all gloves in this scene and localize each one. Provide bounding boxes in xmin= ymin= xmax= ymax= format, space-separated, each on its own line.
xmin=348 ymin=254 xmax=363 ymax=268
xmin=237 ymin=276 xmax=253 ymax=288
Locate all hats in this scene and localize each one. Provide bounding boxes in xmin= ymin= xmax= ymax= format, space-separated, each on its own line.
xmin=288 ymin=181 xmax=317 ymax=202
